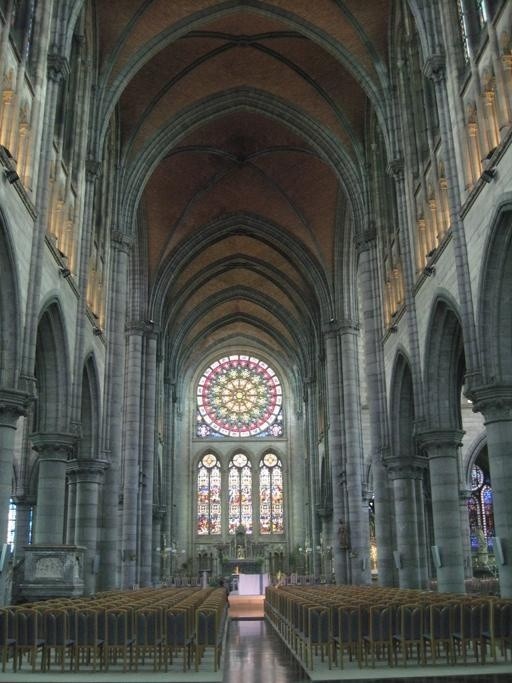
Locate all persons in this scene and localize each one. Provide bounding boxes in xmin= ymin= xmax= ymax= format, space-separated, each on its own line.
xmin=475 ymin=525 xmax=488 ymax=554
xmin=336 ymin=519 xmax=350 ymax=550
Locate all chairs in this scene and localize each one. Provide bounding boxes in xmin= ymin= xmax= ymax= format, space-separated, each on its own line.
xmin=0 ymin=585 xmax=226 ymax=674
xmin=265 ymin=584 xmax=512 ymax=671
